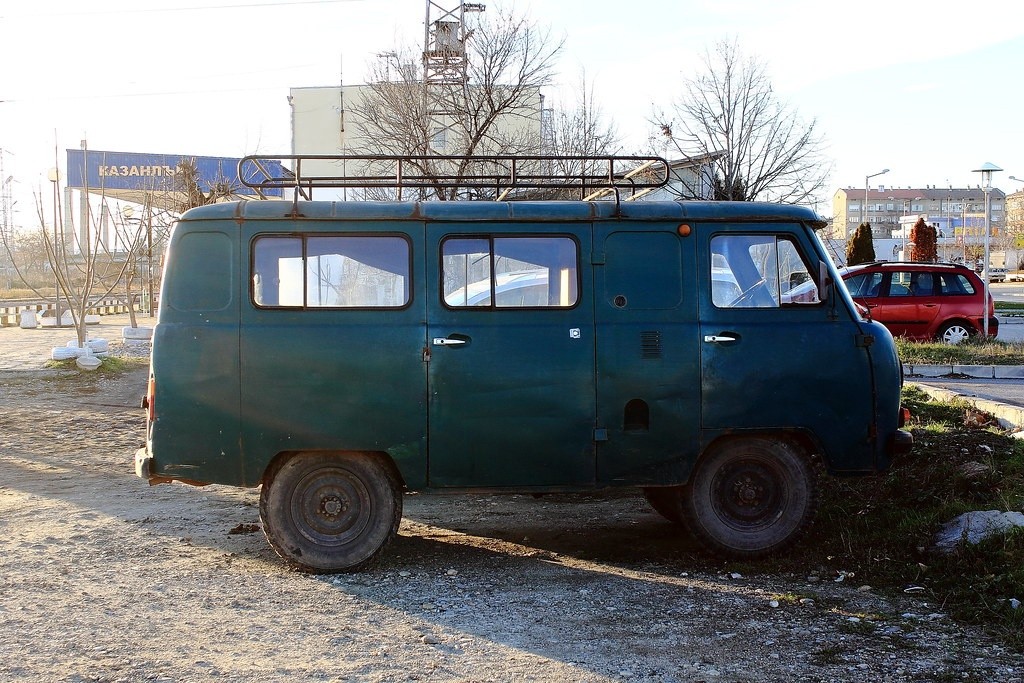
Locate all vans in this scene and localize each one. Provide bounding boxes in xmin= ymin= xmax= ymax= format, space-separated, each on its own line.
xmin=135 ymin=147 xmax=914 ymax=573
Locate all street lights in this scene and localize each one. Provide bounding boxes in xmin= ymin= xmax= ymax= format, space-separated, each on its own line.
xmin=46 ymin=168 xmax=62 ymax=328
xmin=124 ymin=206 xmax=134 ymax=313
xmin=905 ymin=240 xmax=916 ymax=260
xmin=938 ymin=226 xmax=946 ymax=259
xmin=959 ymin=198 xmax=972 ymax=266
xmin=861 ymin=167 xmax=893 ymax=222
xmin=888 ymin=196 xmax=922 ymax=258
xmin=970 ymin=161 xmax=1003 ymax=341
xmin=1009 ymin=176 xmax=1024 ymax=182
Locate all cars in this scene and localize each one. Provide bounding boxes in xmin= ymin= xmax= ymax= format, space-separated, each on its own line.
xmin=443 ymin=266 xmax=753 ymax=310
xmin=969 ymin=259 xmax=984 ymax=271
xmin=773 ymin=260 xmax=999 ymax=344
xmin=953 ymin=256 xmax=969 ymax=265
xmin=981 ymin=265 xmax=1006 ymax=283
